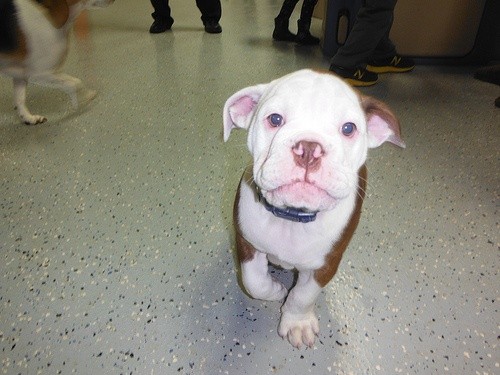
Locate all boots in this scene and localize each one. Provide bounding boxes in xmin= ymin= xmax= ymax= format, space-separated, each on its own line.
xmin=273 ymin=18 xmax=297 ymax=42
xmin=296 ymin=20 xmax=320 ymax=44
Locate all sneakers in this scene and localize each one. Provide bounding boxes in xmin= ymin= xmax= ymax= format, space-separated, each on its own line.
xmin=366 ymin=54 xmax=415 ymax=73
xmin=329 ymin=65 xmax=378 ymax=86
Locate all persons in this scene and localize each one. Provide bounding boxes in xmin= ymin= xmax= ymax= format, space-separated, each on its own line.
xmin=150 ymin=0 xmax=222 ymax=33
xmin=474 ymin=67 xmax=500 ymax=107
xmin=330 ymin=0 xmax=417 ymax=84
xmin=273 ymin=0 xmax=321 ymax=44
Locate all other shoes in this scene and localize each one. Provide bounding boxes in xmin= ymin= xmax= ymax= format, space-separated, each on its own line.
xmin=203 ymin=20 xmax=222 ymax=34
xmin=150 ymin=21 xmax=172 ymax=33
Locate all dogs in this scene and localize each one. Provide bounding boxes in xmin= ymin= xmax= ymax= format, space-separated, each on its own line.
xmin=0 ymin=0 xmax=116 ymax=126
xmin=221 ymin=66 xmax=405 ymax=350
